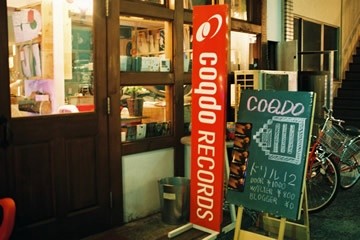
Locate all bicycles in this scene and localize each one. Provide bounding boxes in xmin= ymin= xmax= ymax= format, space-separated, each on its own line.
xmin=300 ymin=105 xmax=360 ymax=215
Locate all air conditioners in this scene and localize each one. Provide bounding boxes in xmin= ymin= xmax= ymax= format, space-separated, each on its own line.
xmin=233 ymin=69 xmax=297 ymax=123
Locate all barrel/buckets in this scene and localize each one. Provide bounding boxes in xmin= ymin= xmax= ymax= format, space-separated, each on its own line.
xmin=157 ymin=177 xmax=191 ymax=225
